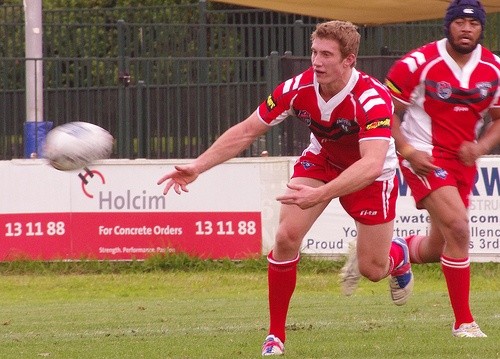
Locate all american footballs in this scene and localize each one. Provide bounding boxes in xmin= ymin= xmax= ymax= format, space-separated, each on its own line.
xmin=41 ymin=120 xmax=114 ymax=171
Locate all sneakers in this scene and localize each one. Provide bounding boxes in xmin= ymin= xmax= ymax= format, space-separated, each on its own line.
xmin=262 ymin=334 xmax=284 ymax=355
xmin=453 ymin=321 xmax=488 ymax=337
xmin=339 ymin=238 xmax=361 ymax=295
xmin=390 ymin=238 xmax=414 ymax=306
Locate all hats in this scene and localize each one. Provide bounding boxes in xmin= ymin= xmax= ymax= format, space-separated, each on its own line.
xmin=445 ymin=0 xmax=486 ymax=32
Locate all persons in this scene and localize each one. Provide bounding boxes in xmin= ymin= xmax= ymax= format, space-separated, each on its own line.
xmin=157 ymin=20 xmax=413 ymax=356
xmin=338 ymin=0 xmax=500 ymax=338
